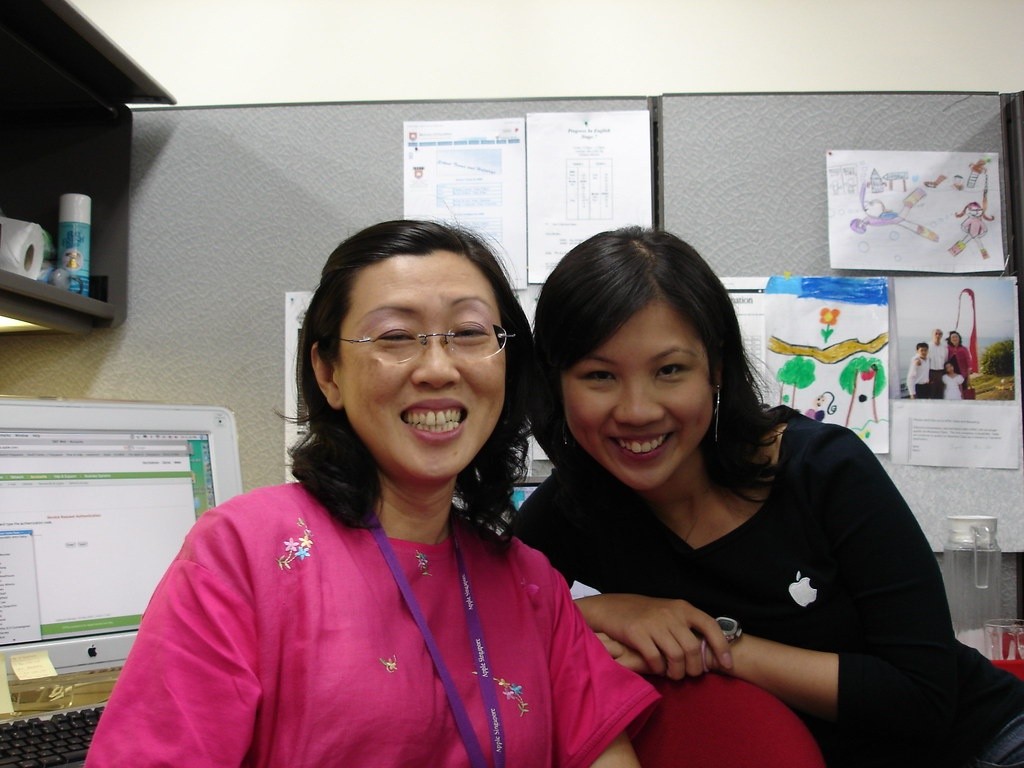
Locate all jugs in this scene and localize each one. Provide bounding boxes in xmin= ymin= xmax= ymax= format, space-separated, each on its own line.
xmin=941 ymin=515 xmax=1003 ymax=649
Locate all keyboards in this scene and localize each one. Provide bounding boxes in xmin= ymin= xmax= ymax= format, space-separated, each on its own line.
xmin=0 ymin=705 xmax=107 ymax=768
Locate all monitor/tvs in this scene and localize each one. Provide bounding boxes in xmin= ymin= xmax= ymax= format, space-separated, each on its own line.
xmin=0 ymin=394 xmax=244 ymax=714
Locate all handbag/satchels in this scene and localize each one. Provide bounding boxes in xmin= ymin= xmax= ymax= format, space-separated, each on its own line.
xmin=962 ymin=374 xmax=976 ymax=399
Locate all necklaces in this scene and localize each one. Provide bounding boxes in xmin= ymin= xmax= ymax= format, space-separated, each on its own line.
xmin=684 ymin=489 xmax=720 ymax=543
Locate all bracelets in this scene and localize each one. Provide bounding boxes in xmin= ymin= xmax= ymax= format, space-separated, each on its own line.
xmin=700 ymin=638 xmax=709 ymax=676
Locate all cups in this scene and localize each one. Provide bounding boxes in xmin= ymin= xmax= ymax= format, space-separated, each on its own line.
xmin=983 ymin=618 xmax=1024 ymax=661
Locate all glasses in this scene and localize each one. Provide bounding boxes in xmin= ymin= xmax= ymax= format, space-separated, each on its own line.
xmin=340 ymin=321 xmax=514 ymax=366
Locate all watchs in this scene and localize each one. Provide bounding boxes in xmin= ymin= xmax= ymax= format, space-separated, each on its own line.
xmin=714 ymin=616 xmax=743 ymax=674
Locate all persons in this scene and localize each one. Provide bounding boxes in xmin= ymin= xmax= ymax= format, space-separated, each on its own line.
xmin=83 ymin=220 xmax=664 ymax=768
xmin=492 ymin=226 xmax=1024 ymax=768
xmin=906 ymin=329 xmax=976 ymax=400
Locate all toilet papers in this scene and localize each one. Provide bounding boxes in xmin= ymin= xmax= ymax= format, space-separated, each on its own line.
xmin=0 ymin=216 xmax=44 ymax=281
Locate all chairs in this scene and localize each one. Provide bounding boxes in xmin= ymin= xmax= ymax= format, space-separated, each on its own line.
xmin=630 ymin=670 xmax=826 ymax=768
